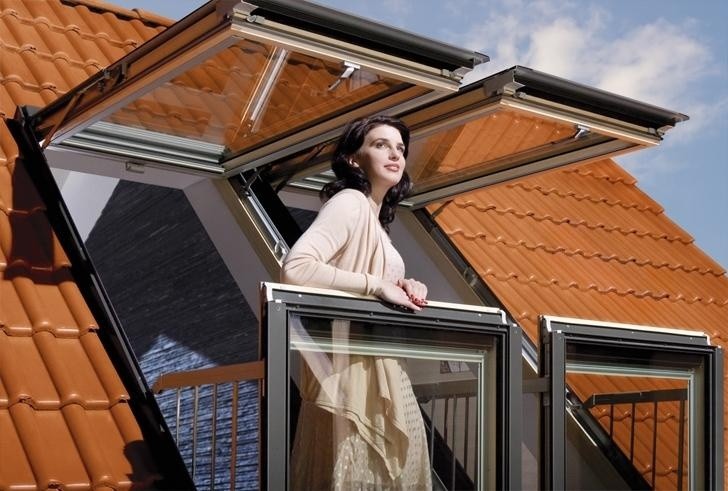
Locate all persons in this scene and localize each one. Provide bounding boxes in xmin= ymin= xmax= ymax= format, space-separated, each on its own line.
xmin=276 ymin=115 xmax=437 ymax=491
